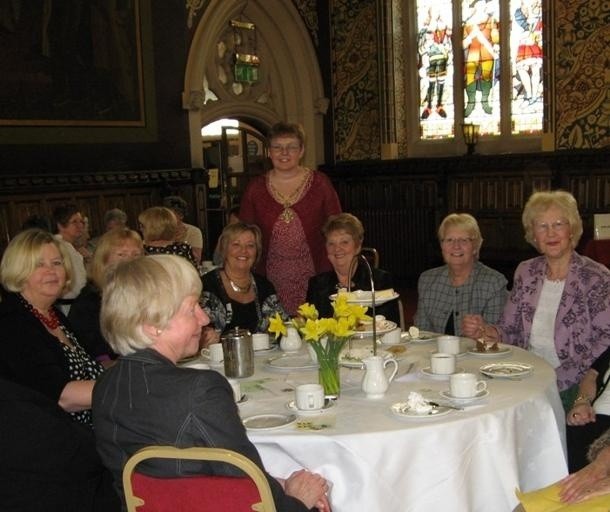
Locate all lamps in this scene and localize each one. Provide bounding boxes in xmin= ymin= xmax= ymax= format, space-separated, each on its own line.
xmin=460 ymin=121 xmax=481 ymax=155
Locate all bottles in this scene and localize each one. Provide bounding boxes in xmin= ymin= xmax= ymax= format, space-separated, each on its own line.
xmin=220 ymin=325 xmax=257 ymax=380
xmin=279 ymin=321 xmax=302 ymax=353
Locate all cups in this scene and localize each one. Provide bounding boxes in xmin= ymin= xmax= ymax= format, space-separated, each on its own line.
xmin=438 ymin=335 xmax=461 ymax=353
xmin=448 ymin=374 xmax=487 ymax=398
xmin=253 ymin=333 xmax=268 ymax=350
xmin=294 ymin=384 xmax=326 ymax=410
xmin=227 ymin=380 xmax=242 ymax=402
xmin=201 ymin=343 xmax=223 ymax=363
xmin=431 ymin=353 xmax=456 ymax=373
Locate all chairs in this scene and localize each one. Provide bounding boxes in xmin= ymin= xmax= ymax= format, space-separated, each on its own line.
xmin=383 ymin=299 xmax=407 ymax=333
xmin=119 ymin=440 xmax=275 ymax=512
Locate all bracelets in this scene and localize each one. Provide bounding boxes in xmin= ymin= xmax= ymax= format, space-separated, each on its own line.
xmin=573 ymin=392 xmax=592 ymax=404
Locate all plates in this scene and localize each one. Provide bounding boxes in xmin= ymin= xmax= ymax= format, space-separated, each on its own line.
xmin=377 ymin=340 xmax=410 ymax=346
xmin=391 ymin=399 xmax=458 ymax=418
xmin=289 ymin=400 xmax=338 ymax=413
xmin=427 ymin=348 xmax=467 ymax=357
xmin=326 ymin=289 xmax=402 ymax=368
xmin=421 ymin=367 xmax=464 ymax=376
xmin=439 ymin=387 xmax=491 ymax=402
xmin=479 ymin=361 xmax=530 ymax=379
xmin=265 ymin=352 xmax=320 ymax=369
xmin=402 ymin=332 xmax=437 ymax=342
xmin=469 ymin=346 xmax=513 ymax=355
xmin=241 ymin=411 xmax=298 ymax=430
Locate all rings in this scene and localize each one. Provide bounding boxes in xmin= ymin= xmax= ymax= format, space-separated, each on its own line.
xmin=572 ymin=414 xmax=577 ymax=417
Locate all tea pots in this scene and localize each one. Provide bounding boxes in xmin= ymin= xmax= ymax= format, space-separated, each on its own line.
xmin=360 ymin=354 xmax=400 ymax=401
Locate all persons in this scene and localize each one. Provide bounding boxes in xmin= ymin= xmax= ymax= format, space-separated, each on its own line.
xmin=513 ymin=428 xmax=610 ymax=512
xmin=1 ymin=196 xmax=400 ymax=512
xmin=566 ymin=345 xmax=610 ymax=454
xmin=412 ymin=213 xmax=511 ymax=339
xmin=238 ymin=121 xmax=342 ymax=320
xmin=463 ymin=191 xmax=610 ymax=392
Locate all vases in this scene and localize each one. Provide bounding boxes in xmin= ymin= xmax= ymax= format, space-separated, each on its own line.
xmin=315 ymin=355 xmax=343 ymax=402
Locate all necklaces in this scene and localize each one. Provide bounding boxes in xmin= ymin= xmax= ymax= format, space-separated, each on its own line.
xmin=270 ymin=167 xmax=309 ymax=223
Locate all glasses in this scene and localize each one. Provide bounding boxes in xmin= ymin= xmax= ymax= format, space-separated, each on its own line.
xmin=265 ymin=144 xmax=303 ymax=152
xmin=532 ymin=219 xmax=569 ymax=232
xmin=63 ymin=216 xmax=86 ymax=226
xmin=439 ymin=235 xmax=476 ymax=246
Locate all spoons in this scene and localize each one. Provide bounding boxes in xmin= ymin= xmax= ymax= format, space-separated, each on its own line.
xmin=429 ymin=402 xmax=463 ymax=410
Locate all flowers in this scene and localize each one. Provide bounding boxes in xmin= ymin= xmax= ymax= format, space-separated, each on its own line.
xmin=266 ymin=285 xmax=376 ymax=399
